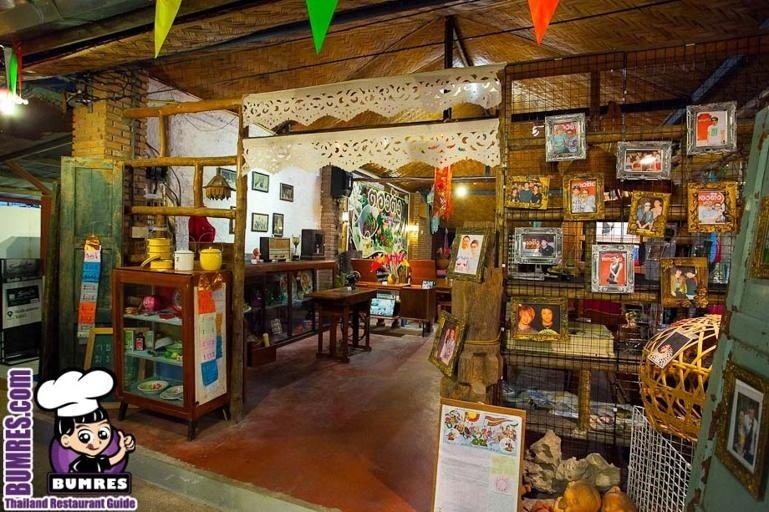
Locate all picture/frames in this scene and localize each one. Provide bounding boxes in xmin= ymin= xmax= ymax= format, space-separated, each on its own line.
xmin=588 ymin=242 xmax=635 ymax=295
xmin=625 ymin=188 xmax=672 ymax=239
xmin=510 ymin=294 xmax=569 ymax=343
xmin=712 ymin=362 xmax=769 ymax=500
xmin=543 ymin=112 xmax=586 ymax=164
xmin=501 ymin=174 xmax=549 ymax=212
xmin=615 ymin=140 xmax=672 ymax=181
xmin=559 ymin=167 xmax=608 ymax=222
xmin=218 ymin=167 xmax=296 ymax=237
xmin=686 ymin=182 xmax=739 ymax=235
xmin=686 ymin=104 xmax=735 ymax=154
xmin=744 ymin=197 xmax=768 ymax=278
xmin=512 ymin=225 xmax=565 ymax=267
xmin=660 ymin=256 xmax=710 ymax=312
xmin=445 ymin=225 xmax=494 ymax=284
xmin=426 ymin=310 xmax=469 ymax=379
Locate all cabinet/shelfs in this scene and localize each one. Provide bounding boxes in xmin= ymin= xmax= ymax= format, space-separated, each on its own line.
xmin=243 ymin=258 xmax=337 ymax=363
xmin=114 ymin=263 xmax=230 ymax=440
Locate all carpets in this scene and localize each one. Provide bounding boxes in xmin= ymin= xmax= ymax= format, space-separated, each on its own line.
xmin=31 ymin=413 xmax=347 ymax=511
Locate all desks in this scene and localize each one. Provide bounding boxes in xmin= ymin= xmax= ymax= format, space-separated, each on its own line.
xmin=354 ymin=280 xmax=436 ymax=337
xmin=305 ymin=287 xmax=378 ymax=360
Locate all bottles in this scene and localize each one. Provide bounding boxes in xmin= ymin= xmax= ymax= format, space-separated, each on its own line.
xmin=135 ymin=332 xmax=147 ymax=351
xmin=407 ymin=272 xmax=412 ymax=287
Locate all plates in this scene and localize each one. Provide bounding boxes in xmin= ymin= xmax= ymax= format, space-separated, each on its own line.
xmin=295 ymin=270 xmax=314 ymax=296
xmin=279 ymin=273 xmax=298 ymax=301
xmin=137 ymin=380 xmax=167 ymax=395
xmin=160 ymin=386 xmax=183 ymax=400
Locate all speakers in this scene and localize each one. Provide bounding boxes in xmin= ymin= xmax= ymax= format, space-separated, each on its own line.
xmin=322 ymin=165 xmax=353 ymax=197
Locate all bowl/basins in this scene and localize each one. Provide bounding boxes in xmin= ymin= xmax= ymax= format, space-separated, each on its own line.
xmin=304 ymin=320 xmax=312 ymax=330
xmin=154 ymin=337 xmax=183 ymax=354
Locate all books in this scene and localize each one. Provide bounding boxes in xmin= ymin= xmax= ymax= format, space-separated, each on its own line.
xmin=503 ymin=322 xmax=646 ymax=429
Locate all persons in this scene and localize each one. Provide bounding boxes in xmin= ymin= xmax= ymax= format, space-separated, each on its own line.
xmin=745 ymin=408 xmax=757 ymax=458
xmin=670 ymin=270 xmax=687 ymax=298
xmin=658 ymin=334 xmax=688 ymax=354
xmin=510 ymin=188 xmax=519 ymax=203
xmin=570 ymin=185 xmax=581 ymax=212
xmin=457 ymin=236 xmax=470 ymax=258
xmin=682 ymin=270 xmax=697 ymax=299
xmin=735 ymin=409 xmax=748 ymax=457
xmin=446 ymin=329 xmax=456 ymax=357
xmin=706 ymin=118 xmax=721 ymax=144
xmin=531 ymin=185 xmax=540 ymax=203
xmin=650 ymin=199 xmax=663 ymax=221
xmin=697 ymin=202 xmax=718 ymax=224
xmin=467 ymin=240 xmax=479 ymax=274
xmin=606 ymin=255 xmax=624 ymax=285
xmin=538 ymin=236 xmax=554 ymax=257
xmin=636 ymin=201 xmax=652 ymax=228
xmin=518 ymin=182 xmax=532 ymax=202
xmin=626 ymin=152 xmax=661 ymax=172
xmin=553 ymin=126 xmax=569 ymax=154
xmin=711 ymin=203 xmax=725 ymax=222
xmin=536 ymin=306 xmax=557 ymax=333
xmin=579 ymin=187 xmax=596 ymax=213
xmin=517 ymin=306 xmax=538 ymax=333
xmin=563 ymin=129 xmax=578 ymax=152
xmin=438 ymin=327 xmax=450 ymax=354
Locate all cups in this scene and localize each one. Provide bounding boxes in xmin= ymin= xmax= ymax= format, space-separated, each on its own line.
xmin=448 ymin=277 xmax=454 ymax=287
xmin=174 ymin=249 xmax=195 ymax=274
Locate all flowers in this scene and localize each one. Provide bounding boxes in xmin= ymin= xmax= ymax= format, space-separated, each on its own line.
xmin=370 ymin=251 xmax=410 ymax=284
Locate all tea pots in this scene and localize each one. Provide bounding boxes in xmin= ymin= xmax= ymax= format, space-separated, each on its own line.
xmin=196 ymin=232 xmax=225 ymax=271
